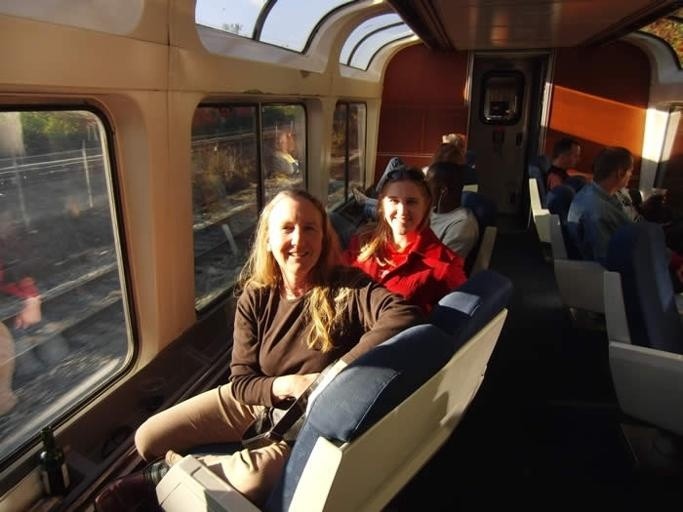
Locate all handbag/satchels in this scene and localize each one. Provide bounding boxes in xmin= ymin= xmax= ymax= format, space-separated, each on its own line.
xmin=267 ymin=404 xmax=306 ymax=441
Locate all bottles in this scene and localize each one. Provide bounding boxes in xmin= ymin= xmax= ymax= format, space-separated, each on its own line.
xmin=38 ymin=427 xmax=73 ymax=497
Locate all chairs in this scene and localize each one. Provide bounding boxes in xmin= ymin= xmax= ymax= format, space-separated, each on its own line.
xmin=154 ymin=160 xmax=683 ymax=512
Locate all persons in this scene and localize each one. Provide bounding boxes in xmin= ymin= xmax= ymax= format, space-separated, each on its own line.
xmin=93 ymin=187 xmax=422 ymax=512
xmin=1 ymin=270 xmax=70 ymax=419
xmin=340 ymin=133 xmax=480 ymax=318
xmin=545 ymin=137 xmax=682 ymax=294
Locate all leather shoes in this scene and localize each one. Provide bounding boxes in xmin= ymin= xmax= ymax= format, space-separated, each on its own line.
xmin=93 ymin=456 xmax=165 ymax=510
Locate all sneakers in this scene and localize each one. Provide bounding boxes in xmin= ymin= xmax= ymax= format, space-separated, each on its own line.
xmin=353 ymin=188 xmax=365 ymax=205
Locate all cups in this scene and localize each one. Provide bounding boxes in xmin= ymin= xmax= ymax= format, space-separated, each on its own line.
xmin=650 ymin=187 xmax=667 ymax=207
xmin=139 ymin=376 xmax=166 ymax=412
